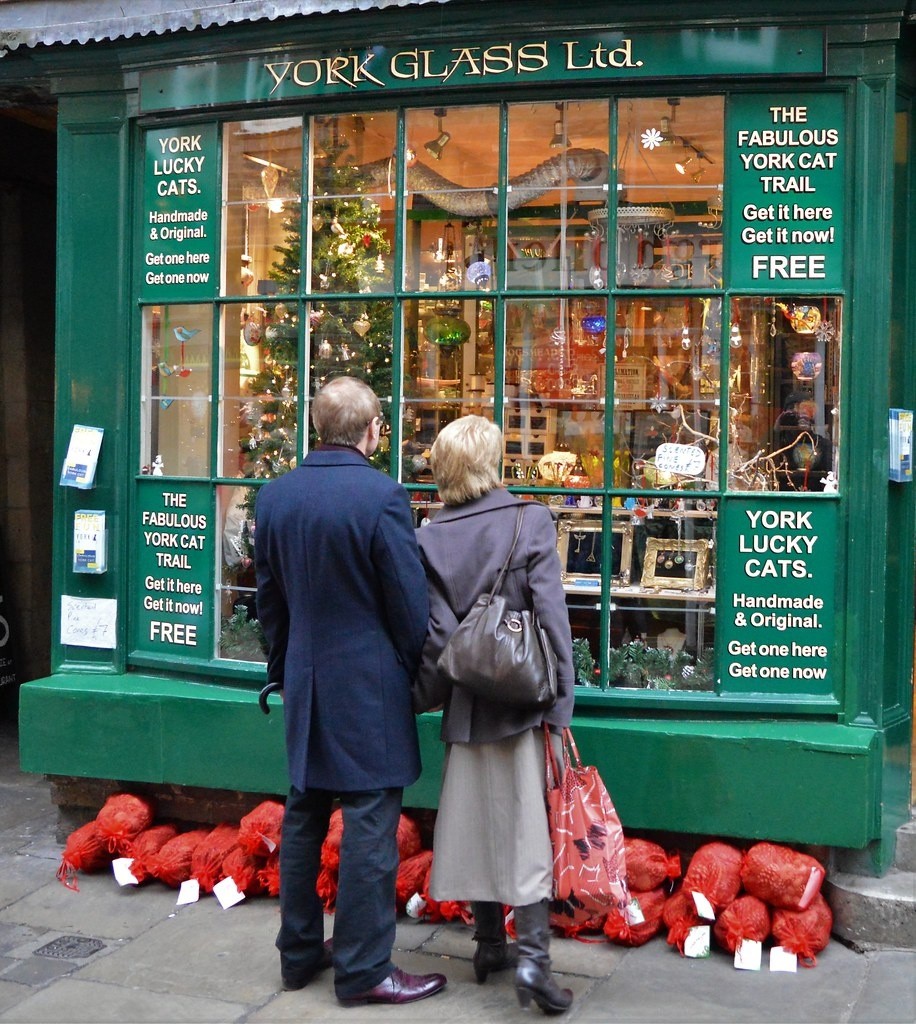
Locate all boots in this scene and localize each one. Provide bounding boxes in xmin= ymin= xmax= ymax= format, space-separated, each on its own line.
xmin=513 ymin=898 xmax=573 ymax=1015
xmin=469 ymin=900 xmax=521 ymax=985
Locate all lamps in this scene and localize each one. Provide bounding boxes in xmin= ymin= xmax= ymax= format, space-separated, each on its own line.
xmin=690 ymin=152 xmax=706 ymax=184
xmin=424 ymin=222 xmax=471 ymax=360
xmin=675 ymin=140 xmax=693 ymax=175
xmin=424 ymin=108 xmax=450 ymax=160
xmin=550 ymin=102 xmax=572 ymax=148
xmin=652 ymin=97 xmax=680 ymax=146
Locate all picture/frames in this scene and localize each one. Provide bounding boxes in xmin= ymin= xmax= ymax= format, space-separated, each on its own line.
xmin=557 ymin=520 xmax=634 ymax=586
xmin=641 ymin=537 xmax=709 ymax=590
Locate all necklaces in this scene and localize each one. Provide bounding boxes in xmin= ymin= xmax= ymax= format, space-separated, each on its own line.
xmin=660 ymin=633 xmax=680 ymax=655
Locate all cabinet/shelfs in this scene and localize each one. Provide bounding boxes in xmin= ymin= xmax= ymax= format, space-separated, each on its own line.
xmin=409 ymin=505 xmax=717 ymax=656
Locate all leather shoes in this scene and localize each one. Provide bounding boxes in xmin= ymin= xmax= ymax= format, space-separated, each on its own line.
xmin=335 ymin=963 xmax=447 ymax=1004
xmin=282 ymin=937 xmax=333 ymax=991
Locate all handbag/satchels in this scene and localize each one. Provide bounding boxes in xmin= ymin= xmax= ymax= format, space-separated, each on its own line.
xmin=436 ymin=593 xmax=558 ymax=712
xmin=544 ymin=720 xmax=632 ymax=924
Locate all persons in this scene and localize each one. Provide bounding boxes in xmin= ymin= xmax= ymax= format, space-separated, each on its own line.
xmin=253 ymin=374 xmax=447 ymax=1005
xmin=412 ymin=415 xmax=575 ymax=1014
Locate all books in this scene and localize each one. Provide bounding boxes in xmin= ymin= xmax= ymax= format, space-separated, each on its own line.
xmin=72 ymin=509 xmax=106 ymax=573
xmin=62 ymin=425 xmax=104 ymax=489
xmin=888 ymin=408 xmax=912 ymax=484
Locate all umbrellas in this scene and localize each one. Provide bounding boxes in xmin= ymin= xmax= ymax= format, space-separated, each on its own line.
xmin=259 ymin=682 xmax=284 ymax=715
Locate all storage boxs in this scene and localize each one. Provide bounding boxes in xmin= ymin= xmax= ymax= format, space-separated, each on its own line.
xmin=598 ymin=363 xmax=656 ymax=410
